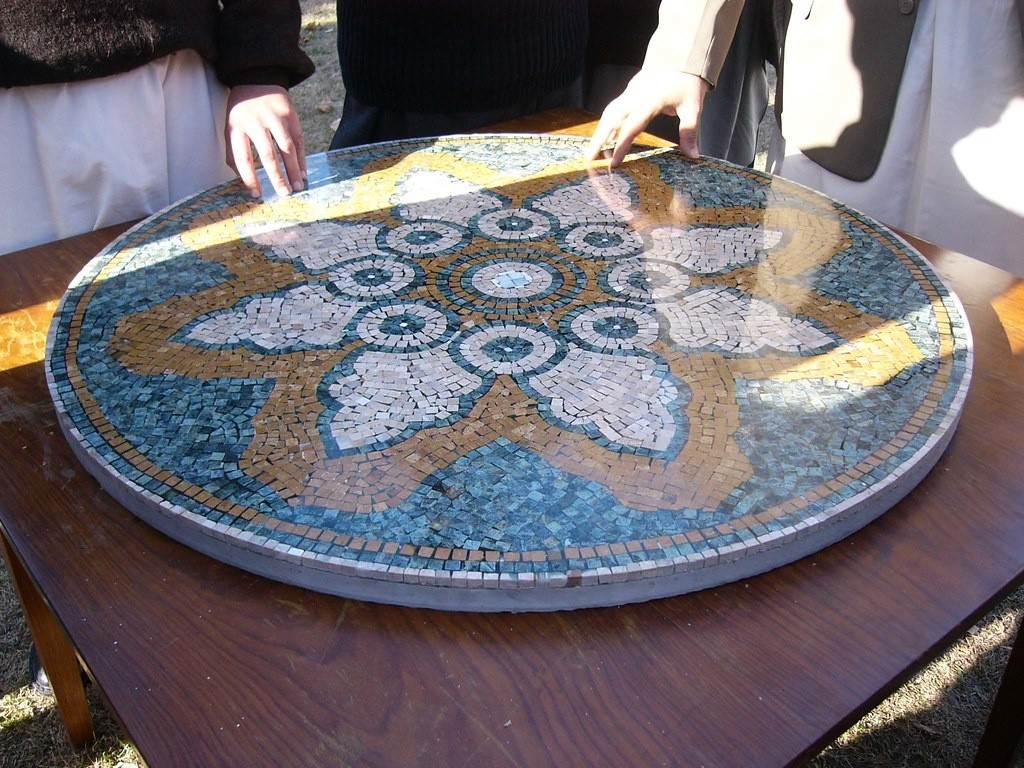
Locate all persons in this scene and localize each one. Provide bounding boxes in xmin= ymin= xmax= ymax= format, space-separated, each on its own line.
xmin=587 ymin=0 xmax=1024 ymax=279
xmin=326 ymin=0 xmax=594 ymax=153
xmin=585 ymin=0 xmax=775 ymax=170
xmin=0 ymin=0 xmax=315 ymax=258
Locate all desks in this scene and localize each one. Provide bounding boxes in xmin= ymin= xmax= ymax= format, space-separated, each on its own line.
xmin=0 ymin=120 xmax=1024 ymax=768
